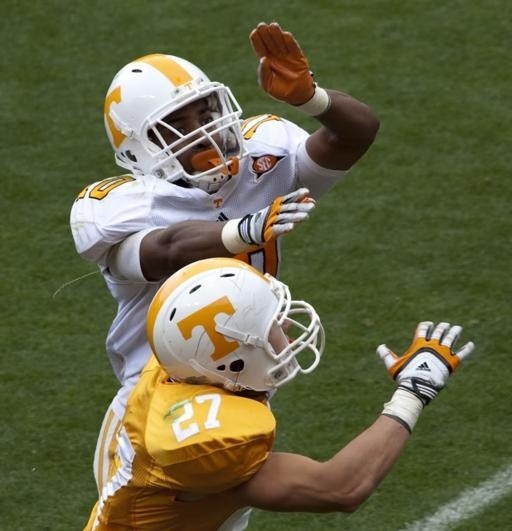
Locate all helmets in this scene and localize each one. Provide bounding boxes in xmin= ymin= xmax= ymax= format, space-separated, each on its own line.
xmin=146 ymin=258 xmax=326 ymax=394
xmin=104 ymin=53 xmax=250 ymax=194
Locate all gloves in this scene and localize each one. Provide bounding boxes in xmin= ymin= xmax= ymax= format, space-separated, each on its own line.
xmin=250 ymin=22 xmax=329 ymax=117
xmin=221 ymin=188 xmax=316 ymax=255
xmin=376 ymin=321 xmax=475 ymax=434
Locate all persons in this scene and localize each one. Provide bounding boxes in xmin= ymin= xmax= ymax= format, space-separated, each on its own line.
xmin=88 ymin=257 xmax=475 ymax=531
xmin=74 ymin=17 xmax=378 ymax=495
